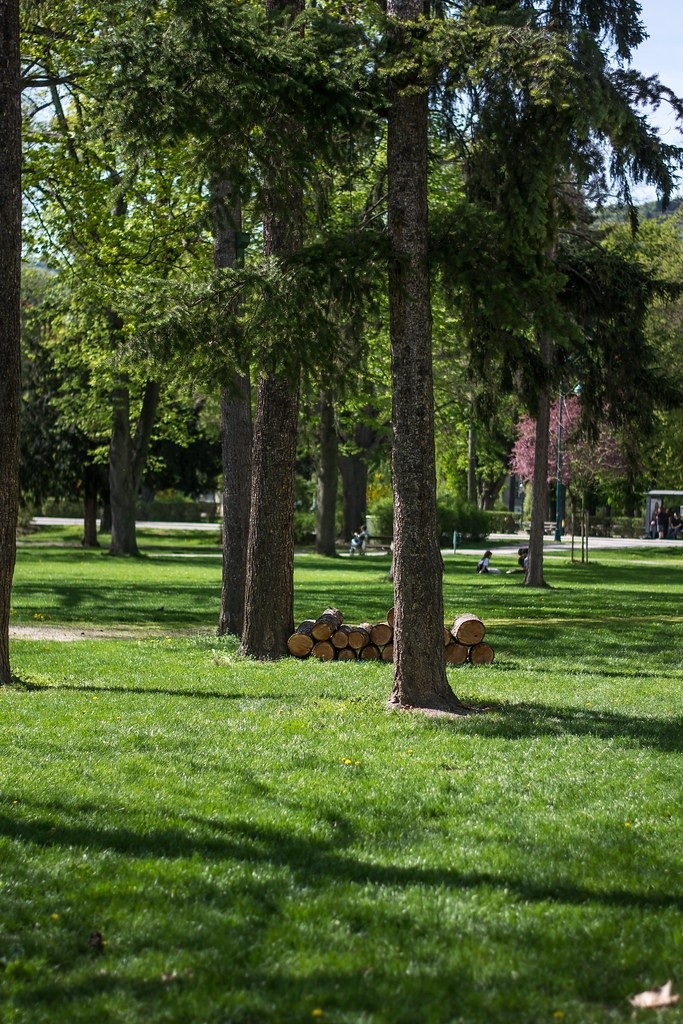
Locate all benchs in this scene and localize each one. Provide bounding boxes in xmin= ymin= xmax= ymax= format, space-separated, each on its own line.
xmin=350 ymin=535 xmax=393 ymax=556
xmin=524 ymin=521 xmax=557 ymax=534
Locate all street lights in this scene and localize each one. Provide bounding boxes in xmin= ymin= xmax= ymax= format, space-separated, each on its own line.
xmin=554 ymin=380 xmax=582 ymax=543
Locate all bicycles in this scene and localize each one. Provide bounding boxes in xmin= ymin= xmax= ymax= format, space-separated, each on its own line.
xmin=349 ymin=535 xmax=365 ymax=558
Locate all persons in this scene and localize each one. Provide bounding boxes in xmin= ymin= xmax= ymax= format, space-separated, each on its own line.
xmin=349 ymin=525 xmax=368 ymax=558
xmin=510 ymin=547 xmax=528 ymax=574
xmin=476 ymin=550 xmax=500 ymax=574
xmin=650 ymin=507 xmax=683 ymax=540
